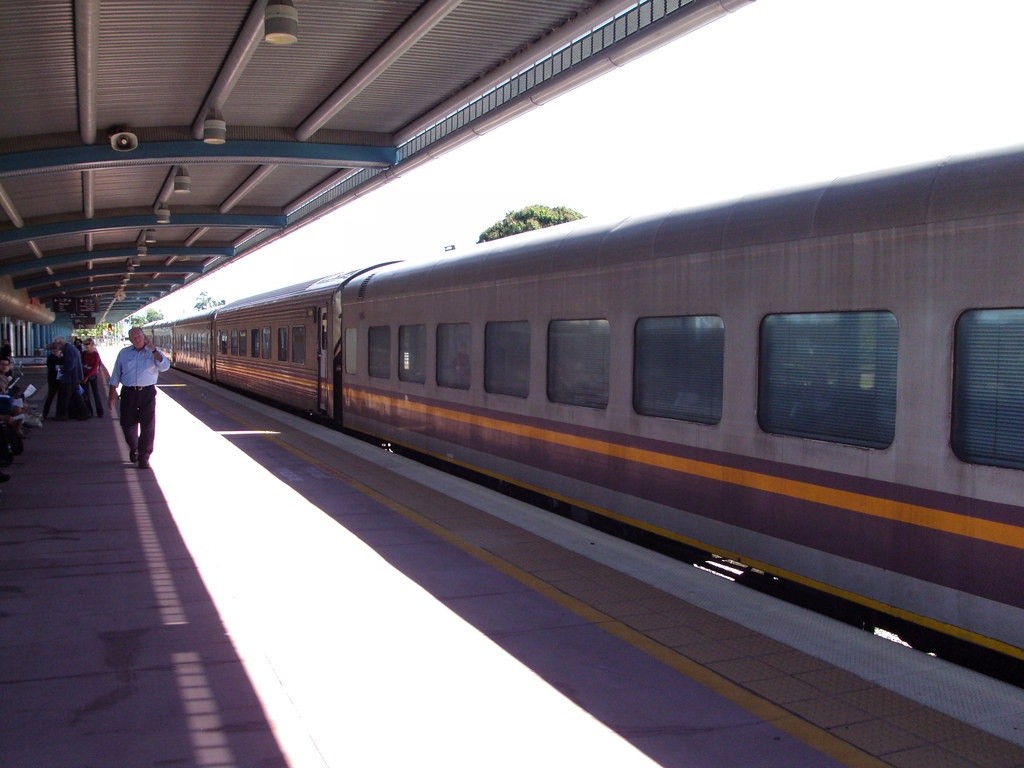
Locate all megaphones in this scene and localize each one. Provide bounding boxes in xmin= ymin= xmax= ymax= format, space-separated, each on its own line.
xmin=111 ymin=132 xmax=138 ymax=152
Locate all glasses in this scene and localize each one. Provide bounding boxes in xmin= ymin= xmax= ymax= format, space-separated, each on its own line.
xmin=84 ymin=343 xmax=90 ymax=345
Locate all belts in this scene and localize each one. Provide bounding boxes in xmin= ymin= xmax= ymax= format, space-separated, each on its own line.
xmin=123 ymin=385 xmax=154 ymax=391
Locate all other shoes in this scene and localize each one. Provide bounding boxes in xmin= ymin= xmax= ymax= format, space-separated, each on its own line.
xmin=139 ymin=456 xmax=149 ymax=469
xmin=130 ymin=448 xmax=138 ymax=462
xmin=51 ymin=416 xmax=68 ymax=420
xmin=0 ymin=470 xmax=10 ymax=482
xmin=16 ymin=427 xmax=23 ymax=435
xmin=78 ymin=414 xmax=92 ymax=420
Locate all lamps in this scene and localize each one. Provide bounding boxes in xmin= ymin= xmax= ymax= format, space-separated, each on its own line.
xmin=175 ymin=167 xmax=191 ymax=193
xmin=264 ymin=0 xmax=298 ymax=46
xmin=132 ymin=257 xmax=141 ymax=267
xmin=156 ymin=202 xmax=170 ymax=224
xmin=136 ymin=243 xmax=147 ymax=256
xmin=145 ymin=229 xmax=158 ymax=243
xmin=204 ymin=109 xmax=226 ymax=145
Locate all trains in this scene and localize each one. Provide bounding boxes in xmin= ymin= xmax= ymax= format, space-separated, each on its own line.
xmin=135 ymin=144 xmax=1023 ymax=664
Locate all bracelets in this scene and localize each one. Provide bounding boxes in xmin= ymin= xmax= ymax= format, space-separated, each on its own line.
xmin=152 ymin=348 xmax=156 ymax=353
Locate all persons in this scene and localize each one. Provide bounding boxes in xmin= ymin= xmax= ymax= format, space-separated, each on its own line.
xmin=107 ymin=327 xmax=170 ymax=469
xmin=0 ymin=339 xmax=28 ymax=483
xmin=40 ymin=338 xmax=105 ymax=423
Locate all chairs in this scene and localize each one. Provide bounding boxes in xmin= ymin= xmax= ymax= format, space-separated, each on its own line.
xmin=14 ymin=361 xmax=24 ymax=376
